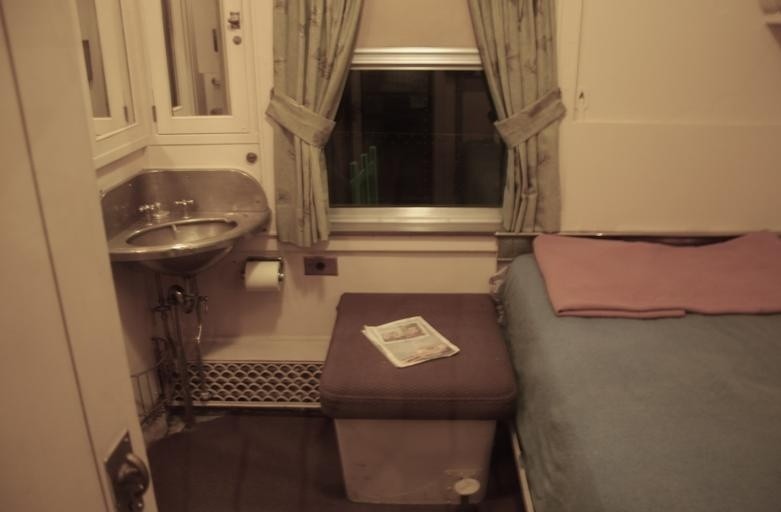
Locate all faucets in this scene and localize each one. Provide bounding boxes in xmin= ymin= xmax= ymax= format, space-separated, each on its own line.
xmin=149 ymin=200 xmax=171 ymax=218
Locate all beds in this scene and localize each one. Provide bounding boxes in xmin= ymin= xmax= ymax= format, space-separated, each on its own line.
xmin=491 ymin=229 xmax=781 ymax=512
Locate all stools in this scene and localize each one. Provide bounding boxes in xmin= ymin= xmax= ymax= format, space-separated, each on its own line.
xmin=316 ymin=291 xmax=516 ymax=508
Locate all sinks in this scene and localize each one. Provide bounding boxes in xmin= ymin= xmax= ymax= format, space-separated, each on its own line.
xmin=122 ymin=215 xmax=239 ymax=248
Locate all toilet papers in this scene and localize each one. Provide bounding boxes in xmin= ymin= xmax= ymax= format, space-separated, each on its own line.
xmin=244 ymin=261 xmax=282 ymax=293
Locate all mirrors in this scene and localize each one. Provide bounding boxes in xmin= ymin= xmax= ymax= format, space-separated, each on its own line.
xmin=75 ymin=0 xmax=147 ymax=158
xmin=146 ymin=1 xmax=249 ymax=134
